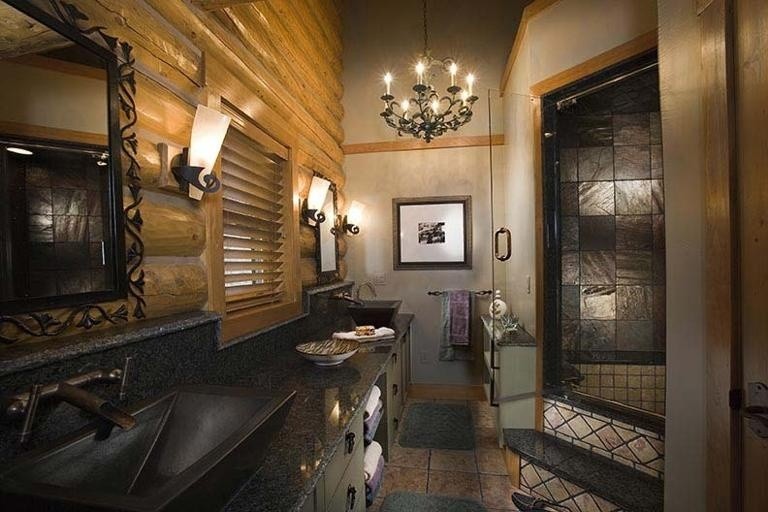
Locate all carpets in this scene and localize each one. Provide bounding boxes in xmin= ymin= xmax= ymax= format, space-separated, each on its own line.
xmin=380 ymin=402 xmax=490 ymax=511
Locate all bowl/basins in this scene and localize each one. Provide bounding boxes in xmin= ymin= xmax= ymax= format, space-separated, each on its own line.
xmin=294 ymin=336 xmax=359 ymax=368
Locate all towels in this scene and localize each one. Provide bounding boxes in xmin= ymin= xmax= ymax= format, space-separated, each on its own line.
xmin=439 ymin=288 xmax=476 ymax=362
xmin=332 ymin=327 xmax=395 ymax=344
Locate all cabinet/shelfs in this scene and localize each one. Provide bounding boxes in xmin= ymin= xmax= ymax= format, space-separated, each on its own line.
xmin=479 ymin=313 xmax=536 ymax=449
xmin=299 ymin=326 xmax=412 ymax=512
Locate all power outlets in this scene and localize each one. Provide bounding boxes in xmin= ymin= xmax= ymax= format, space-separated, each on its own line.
xmin=374 ymin=272 xmax=387 ymax=286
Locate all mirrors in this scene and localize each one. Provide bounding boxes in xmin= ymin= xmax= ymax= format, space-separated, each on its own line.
xmin=0 ymin=0 xmax=132 ymax=317
xmin=314 ymin=169 xmax=339 ymax=277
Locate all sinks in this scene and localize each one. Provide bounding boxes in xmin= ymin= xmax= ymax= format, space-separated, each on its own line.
xmin=350 ymin=300 xmax=402 ymax=328
xmin=1 ymin=380 xmax=297 ymax=511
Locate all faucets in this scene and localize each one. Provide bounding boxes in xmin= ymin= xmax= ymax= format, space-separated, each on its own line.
xmin=356 ymin=280 xmax=378 ymax=300
xmin=330 ymin=289 xmax=367 ymax=307
xmin=57 ymin=381 xmax=137 ymax=432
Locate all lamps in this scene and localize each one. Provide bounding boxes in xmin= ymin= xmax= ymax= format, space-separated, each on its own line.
xmin=378 ymin=1 xmax=479 ymax=143
xmin=299 ymin=175 xmax=363 ymax=237
xmin=169 ymin=103 xmax=232 ymax=201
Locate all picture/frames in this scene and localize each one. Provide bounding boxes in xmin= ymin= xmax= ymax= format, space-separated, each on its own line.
xmin=391 ymin=195 xmax=473 ymax=272
